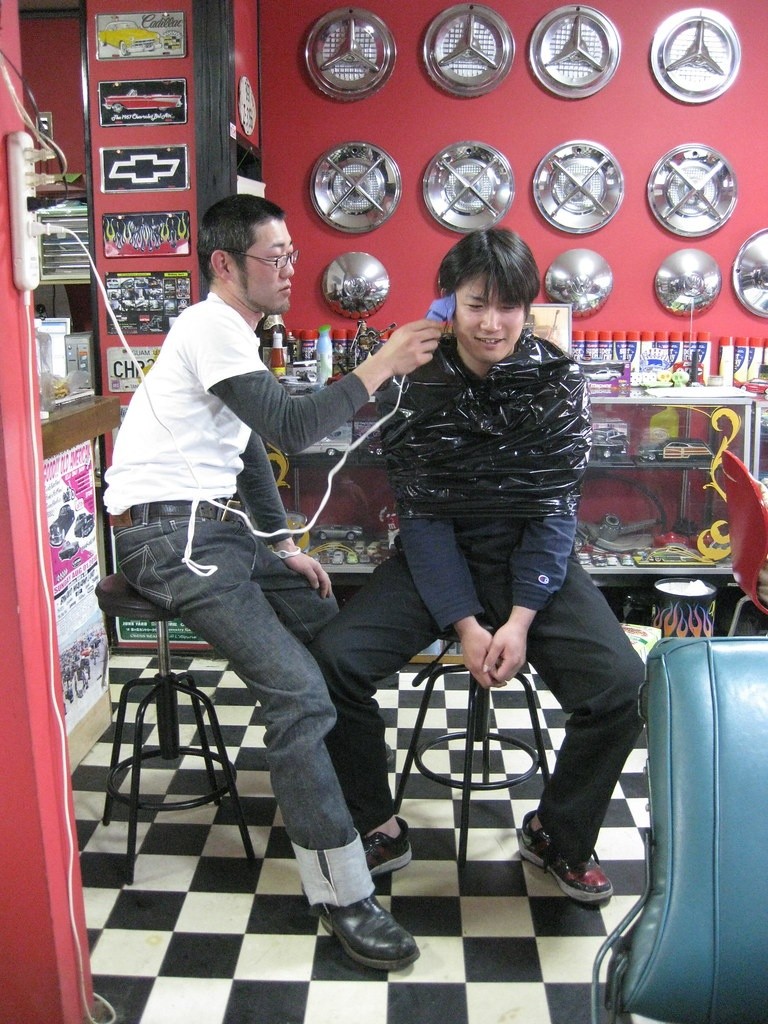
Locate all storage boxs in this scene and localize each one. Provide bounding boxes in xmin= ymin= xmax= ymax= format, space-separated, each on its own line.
xmin=580 ymin=362 xmax=631 ymax=387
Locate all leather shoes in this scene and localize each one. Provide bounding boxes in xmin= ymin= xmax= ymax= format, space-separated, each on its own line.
xmin=320 ymin=892 xmax=421 ymax=971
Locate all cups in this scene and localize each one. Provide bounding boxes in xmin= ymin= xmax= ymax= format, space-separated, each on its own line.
xmin=292 ymin=360 xmax=317 ymax=382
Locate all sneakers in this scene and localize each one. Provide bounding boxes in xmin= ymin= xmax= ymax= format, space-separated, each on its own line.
xmin=360 ymin=816 xmax=413 ymax=876
xmin=517 ymin=810 xmax=614 ymax=903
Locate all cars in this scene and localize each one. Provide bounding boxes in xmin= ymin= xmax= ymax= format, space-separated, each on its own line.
xmin=590 ymin=427 xmax=714 ymax=463
xmin=313 ymin=540 xmax=397 ymax=566
xmin=278 ymin=372 xmax=344 ymax=395
xmin=296 ymin=429 xmax=382 ymax=458
xmin=582 ymin=368 xmax=622 ymax=383
xmin=733 ymin=378 xmax=768 ymax=395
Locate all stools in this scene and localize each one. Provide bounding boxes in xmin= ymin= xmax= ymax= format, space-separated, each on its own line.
xmin=388 ymin=618 xmax=560 ymax=874
xmin=91 ymin=569 xmax=265 ymax=887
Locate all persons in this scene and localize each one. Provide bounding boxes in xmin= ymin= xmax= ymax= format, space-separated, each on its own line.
xmin=103 ymin=194 xmax=443 ymax=970
xmin=308 ymin=227 xmax=646 ymax=901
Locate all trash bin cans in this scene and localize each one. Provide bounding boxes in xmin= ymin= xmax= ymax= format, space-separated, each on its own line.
xmin=653 ymin=578 xmax=720 ymax=638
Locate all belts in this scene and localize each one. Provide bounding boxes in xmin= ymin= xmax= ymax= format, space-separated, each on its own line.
xmin=130 ymin=498 xmax=232 ymax=520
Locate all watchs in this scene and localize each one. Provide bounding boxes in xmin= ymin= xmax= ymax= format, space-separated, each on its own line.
xmin=273 ymin=546 xmax=302 ymax=559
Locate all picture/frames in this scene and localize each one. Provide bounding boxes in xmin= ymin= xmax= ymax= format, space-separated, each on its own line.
xmin=529 ymin=303 xmax=573 ymax=355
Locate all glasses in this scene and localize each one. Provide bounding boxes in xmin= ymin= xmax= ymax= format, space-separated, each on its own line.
xmin=216 ymin=248 xmax=300 ymax=269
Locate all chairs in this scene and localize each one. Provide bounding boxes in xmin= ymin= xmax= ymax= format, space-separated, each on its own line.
xmin=588 ymin=633 xmax=768 ymax=1024
xmin=718 ymin=446 xmax=768 ymax=637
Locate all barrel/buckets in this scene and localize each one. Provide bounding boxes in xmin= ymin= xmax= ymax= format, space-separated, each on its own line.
xmin=653 ymin=578 xmax=717 ymax=639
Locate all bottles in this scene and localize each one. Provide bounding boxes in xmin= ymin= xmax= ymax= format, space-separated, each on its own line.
xmin=286 ymin=324 xmax=393 ymax=384
xmin=271 ymin=333 xmax=286 ymax=383
xmin=572 ymin=330 xmax=768 ymax=394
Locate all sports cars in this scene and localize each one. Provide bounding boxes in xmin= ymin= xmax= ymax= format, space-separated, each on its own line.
xmin=102 ymin=88 xmax=184 ymax=114
xmin=97 ymin=19 xmax=163 ymax=57
xmin=309 ymin=523 xmax=364 ymax=542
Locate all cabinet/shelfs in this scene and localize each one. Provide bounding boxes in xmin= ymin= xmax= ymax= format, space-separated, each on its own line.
xmin=579 ymin=378 xmax=756 ymax=591
xmin=292 ymin=355 xmax=403 ymax=584
